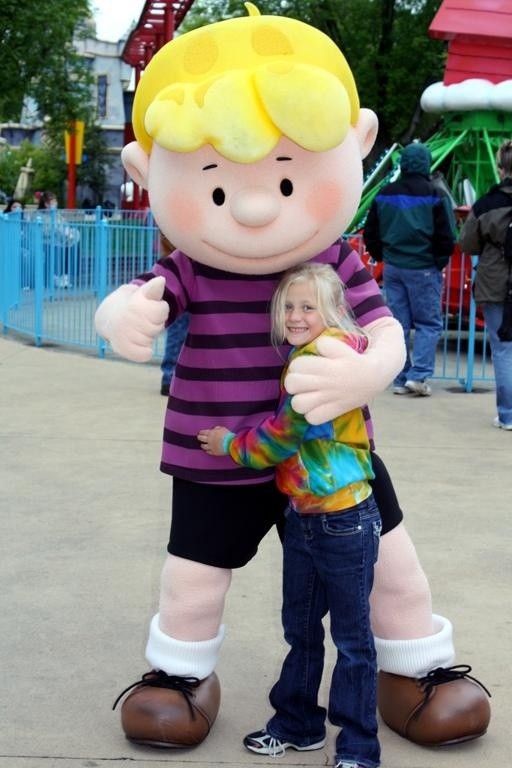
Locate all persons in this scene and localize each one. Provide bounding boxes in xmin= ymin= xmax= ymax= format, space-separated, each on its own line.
xmin=459 ymin=141 xmax=511 ymax=430
xmin=92 ymin=1 xmax=491 ymax=749
xmin=198 ymin=262 xmax=381 ymax=768
xmin=363 ymin=144 xmax=457 ymax=396
xmin=3 ymin=191 xmax=73 ymax=292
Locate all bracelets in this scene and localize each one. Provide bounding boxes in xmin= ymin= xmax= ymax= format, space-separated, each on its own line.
xmin=223 ymin=432 xmax=234 ymax=453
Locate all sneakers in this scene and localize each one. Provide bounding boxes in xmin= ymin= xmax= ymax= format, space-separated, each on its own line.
xmin=243 ymin=728 xmax=327 ymax=757
xmin=492 ymin=417 xmax=512 ymax=430
xmin=405 ymin=380 xmax=432 ymax=396
xmin=392 ymin=385 xmax=409 ymax=395
